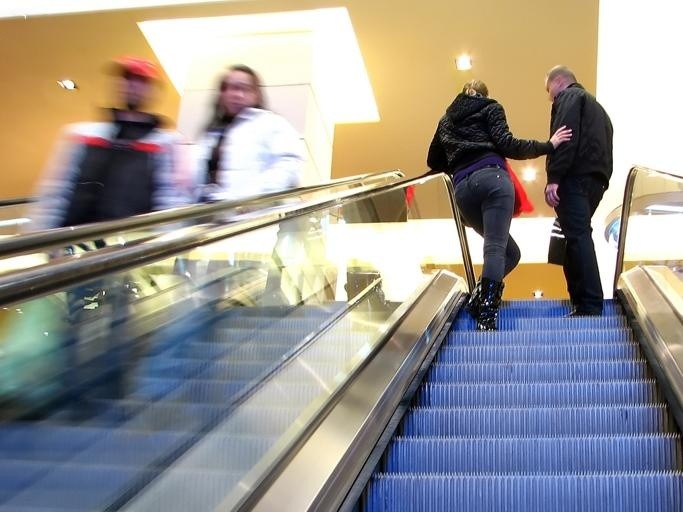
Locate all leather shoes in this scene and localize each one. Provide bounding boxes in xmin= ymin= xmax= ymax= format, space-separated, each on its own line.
xmin=567 ymin=309 xmax=602 ymax=316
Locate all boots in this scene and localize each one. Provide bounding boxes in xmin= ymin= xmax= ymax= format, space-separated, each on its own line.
xmin=455 ymin=275 xmax=504 ymax=332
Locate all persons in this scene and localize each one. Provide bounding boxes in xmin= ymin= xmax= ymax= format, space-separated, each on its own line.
xmin=544 ymin=65 xmax=614 ymax=317
xmin=20 ymin=56 xmax=191 ymax=427
xmin=181 ymin=65 xmax=311 ymax=311
xmin=426 ymin=79 xmax=573 ymax=331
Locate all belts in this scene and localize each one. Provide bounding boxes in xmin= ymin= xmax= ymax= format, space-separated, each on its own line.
xmin=468 ymin=164 xmax=505 ymax=175
